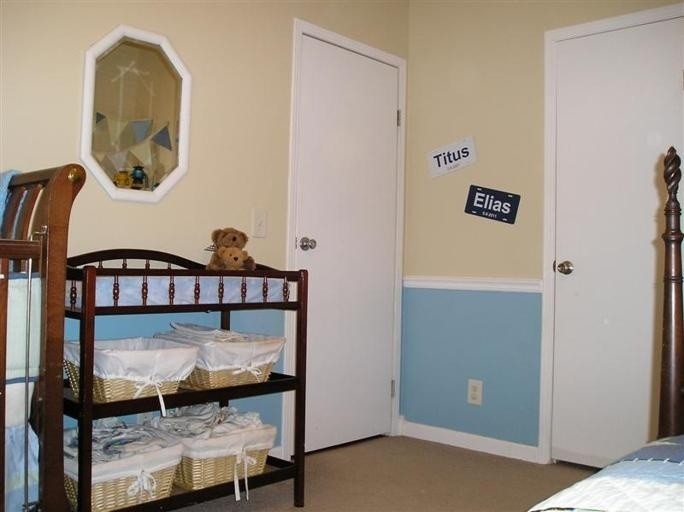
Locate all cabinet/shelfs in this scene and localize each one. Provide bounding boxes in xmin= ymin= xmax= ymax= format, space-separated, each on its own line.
xmin=64 ymin=249 xmax=307 ymax=511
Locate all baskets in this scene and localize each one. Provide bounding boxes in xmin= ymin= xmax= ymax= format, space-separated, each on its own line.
xmin=59 ymin=331 xmax=197 ymax=402
xmin=63 ymin=424 xmax=184 ymax=512
xmin=144 ymin=424 xmax=276 ymax=491
xmin=155 ymin=332 xmax=286 ymax=390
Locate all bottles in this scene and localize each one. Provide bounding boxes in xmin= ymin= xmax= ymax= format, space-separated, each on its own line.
xmin=132 ymin=166 xmax=143 ymax=182
xmin=115 ymin=171 xmax=129 ymax=187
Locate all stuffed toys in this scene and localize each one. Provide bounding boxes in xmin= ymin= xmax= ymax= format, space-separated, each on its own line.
xmin=203 ymin=227 xmax=257 ymax=271
xmin=216 ymin=247 xmax=248 ymax=271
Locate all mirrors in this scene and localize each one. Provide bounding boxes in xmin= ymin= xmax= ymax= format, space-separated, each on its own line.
xmin=80 ymin=24 xmax=193 ymax=207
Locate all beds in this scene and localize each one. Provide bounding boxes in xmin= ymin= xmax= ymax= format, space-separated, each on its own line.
xmin=0 ymin=163 xmax=86 ymax=511
xmin=526 ymin=145 xmax=684 ymax=512
